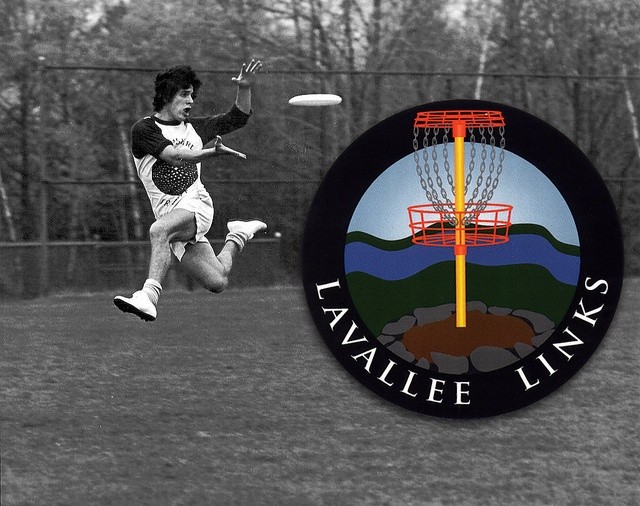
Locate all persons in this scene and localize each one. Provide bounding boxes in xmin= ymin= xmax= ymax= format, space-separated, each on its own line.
xmin=113 ymin=59 xmax=267 ymax=322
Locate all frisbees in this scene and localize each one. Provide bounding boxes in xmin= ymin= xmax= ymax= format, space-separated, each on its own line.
xmin=288 ymin=94 xmax=342 ymax=106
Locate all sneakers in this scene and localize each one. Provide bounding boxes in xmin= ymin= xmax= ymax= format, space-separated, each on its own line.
xmin=227 ymin=220 xmax=267 ymax=240
xmin=114 ymin=290 xmax=158 ymax=322
